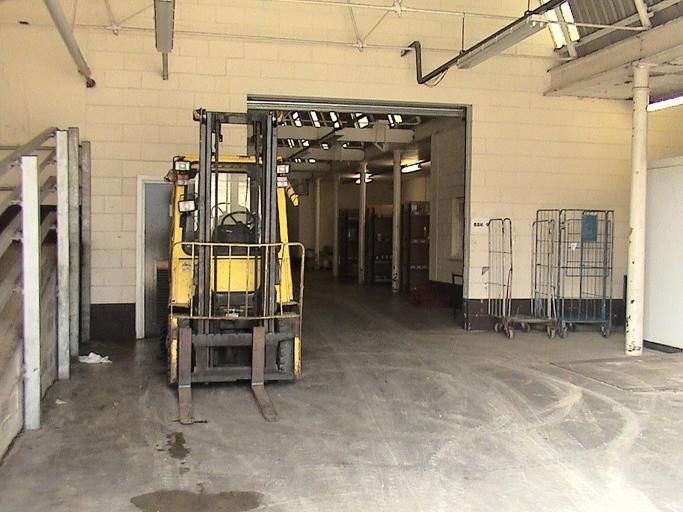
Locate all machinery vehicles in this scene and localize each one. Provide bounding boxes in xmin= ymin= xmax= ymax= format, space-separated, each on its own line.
xmin=161 ymin=107 xmax=302 ymax=426
